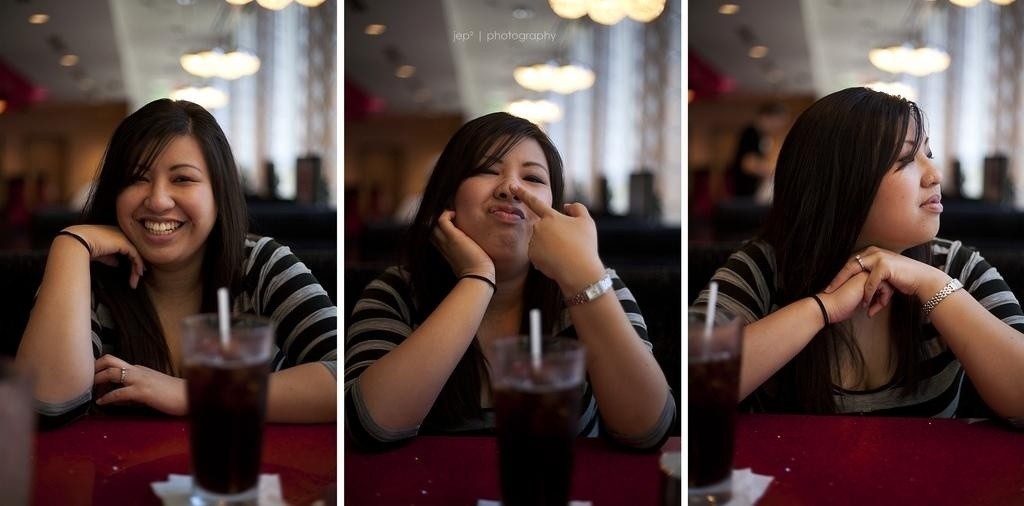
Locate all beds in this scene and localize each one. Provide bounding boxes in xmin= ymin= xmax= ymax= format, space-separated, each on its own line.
xmin=30 ymin=419 xmax=337 ymax=505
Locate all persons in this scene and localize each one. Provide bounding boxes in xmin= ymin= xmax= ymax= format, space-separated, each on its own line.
xmin=344 ymin=111 xmax=675 ymax=453
xmin=688 ymin=86 xmax=1024 ymax=434
xmin=10 ymin=97 xmax=336 ymax=425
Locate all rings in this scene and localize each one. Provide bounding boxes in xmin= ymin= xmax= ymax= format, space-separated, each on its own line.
xmin=120 ymin=367 xmax=127 ymax=384
xmin=855 ymin=253 xmax=866 ymax=271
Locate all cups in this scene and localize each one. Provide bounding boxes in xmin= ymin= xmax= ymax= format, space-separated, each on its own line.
xmin=688 ymin=307 xmax=742 ymax=506
xmin=178 ymin=316 xmax=277 ymax=506
xmin=0 ymin=363 xmax=37 ymax=506
xmin=488 ymin=338 xmax=587 ymax=505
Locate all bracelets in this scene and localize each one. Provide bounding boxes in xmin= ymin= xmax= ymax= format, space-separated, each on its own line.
xmin=52 ymin=229 xmax=94 ymax=255
xmin=916 ymin=280 xmax=964 ymax=316
xmin=812 ymin=292 xmax=832 ymax=329
xmin=460 ymin=272 xmax=497 ymax=293
xmin=559 ymin=272 xmax=617 ymax=308
